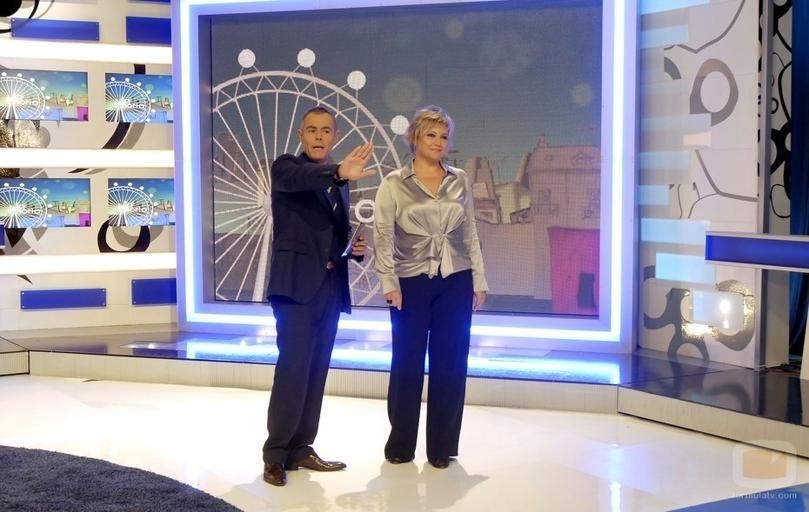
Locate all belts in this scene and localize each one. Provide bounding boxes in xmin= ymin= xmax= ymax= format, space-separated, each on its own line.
xmin=327 ymin=262 xmax=338 ymax=271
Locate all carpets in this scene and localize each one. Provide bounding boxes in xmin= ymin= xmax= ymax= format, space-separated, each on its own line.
xmin=0 ymin=445 xmax=246 ymax=512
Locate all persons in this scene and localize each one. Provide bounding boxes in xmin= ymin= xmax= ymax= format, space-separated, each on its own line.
xmin=373 ymin=104 xmax=490 ymax=470
xmin=261 ymin=106 xmax=377 ymax=487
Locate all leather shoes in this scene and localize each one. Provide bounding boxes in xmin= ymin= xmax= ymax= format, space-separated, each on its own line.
xmin=431 ymin=456 xmax=450 ymax=469
xmin=285 ymin=447 xmax=346 ymax=472
xmin=263 ymin=462 xmax=287 ymax=486
xmin=390 ymin=457 xmax=405 ymax=463
